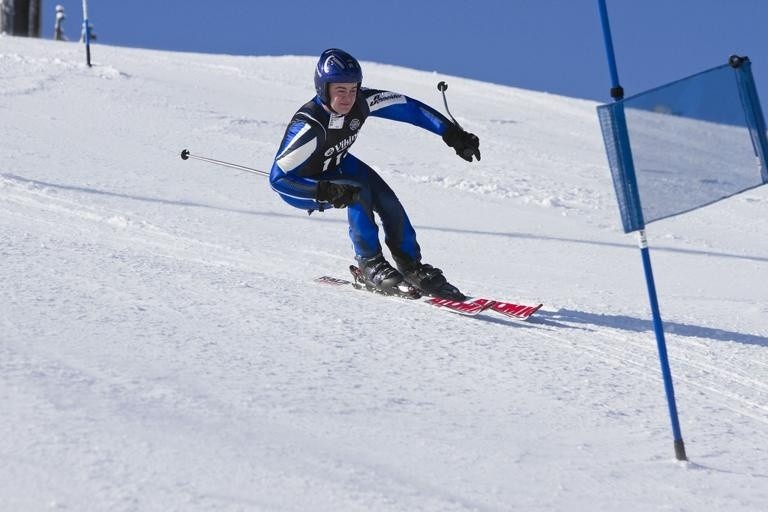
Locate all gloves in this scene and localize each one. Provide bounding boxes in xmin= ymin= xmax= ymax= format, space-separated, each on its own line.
xmin=317 ymin=181 xmax=360 ymax=208
xmin=443 ymin=127 xmax=481 ymax=162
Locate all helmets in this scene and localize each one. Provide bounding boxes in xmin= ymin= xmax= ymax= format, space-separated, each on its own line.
xmin=315 ymin=48 xmax=361 ymax=104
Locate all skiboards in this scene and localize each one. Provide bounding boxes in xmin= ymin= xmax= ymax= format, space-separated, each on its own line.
xmin=315 ymin=273 xmax=543 ymax=321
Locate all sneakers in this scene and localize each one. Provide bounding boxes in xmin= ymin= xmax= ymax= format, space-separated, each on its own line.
xmin=354 ymin=256 xmax=402 ymax=288
xmin=393 ymin=254 xmax=446 ymax=290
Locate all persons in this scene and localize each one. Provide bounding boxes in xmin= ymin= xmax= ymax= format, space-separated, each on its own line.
xmin=269 ymin=48 xmax=480 ymax=296
